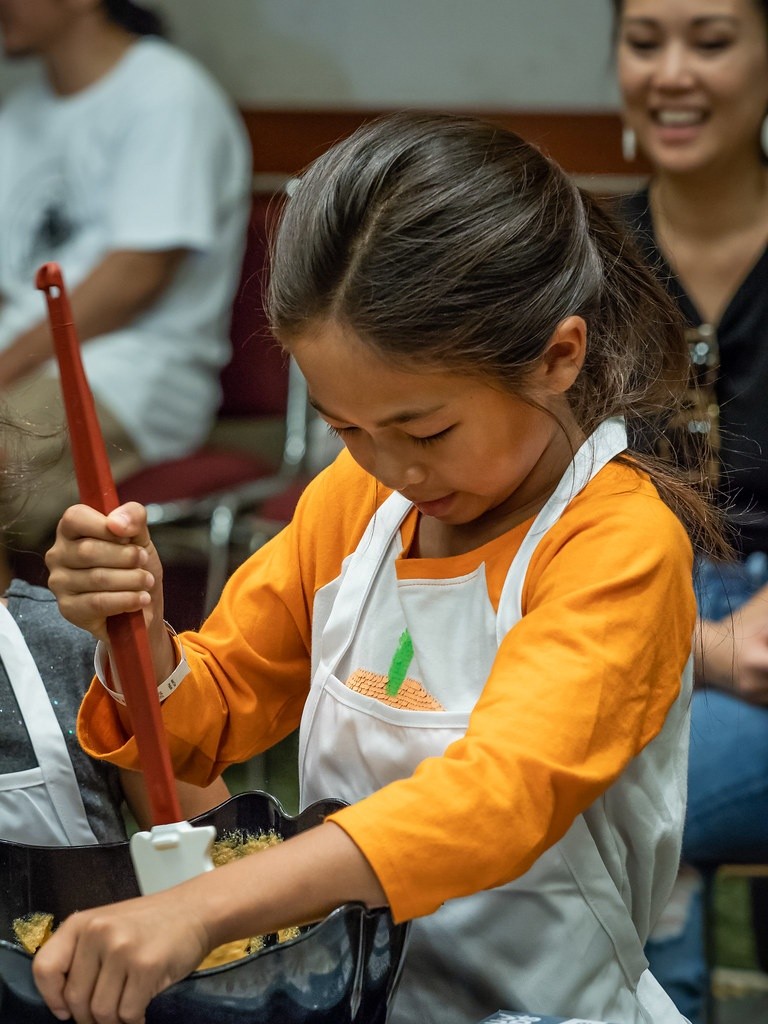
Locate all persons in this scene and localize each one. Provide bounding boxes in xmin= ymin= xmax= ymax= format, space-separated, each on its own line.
xmin=29 ymin=110 xmax=696 ymax=1024
xmin=1 ymin=514 xmax=233 ymax=847
xmin=577 ymin=1 xmax=768 ymax=1024
xmin=0 ymin=0 xmax=254 ymax=564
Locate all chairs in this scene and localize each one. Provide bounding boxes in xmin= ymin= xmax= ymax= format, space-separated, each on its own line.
xmin=102 ymin=174 xmax=315 ymax=630
xmin=227 ymin=407 xmax=354 ymax=793
xmin=575 ymin=177 xmax=766 ymax=1023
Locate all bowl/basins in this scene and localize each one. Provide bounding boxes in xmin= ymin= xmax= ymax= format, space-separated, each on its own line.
xmin=1 ymin=790 xmax=412 ymax=1024
